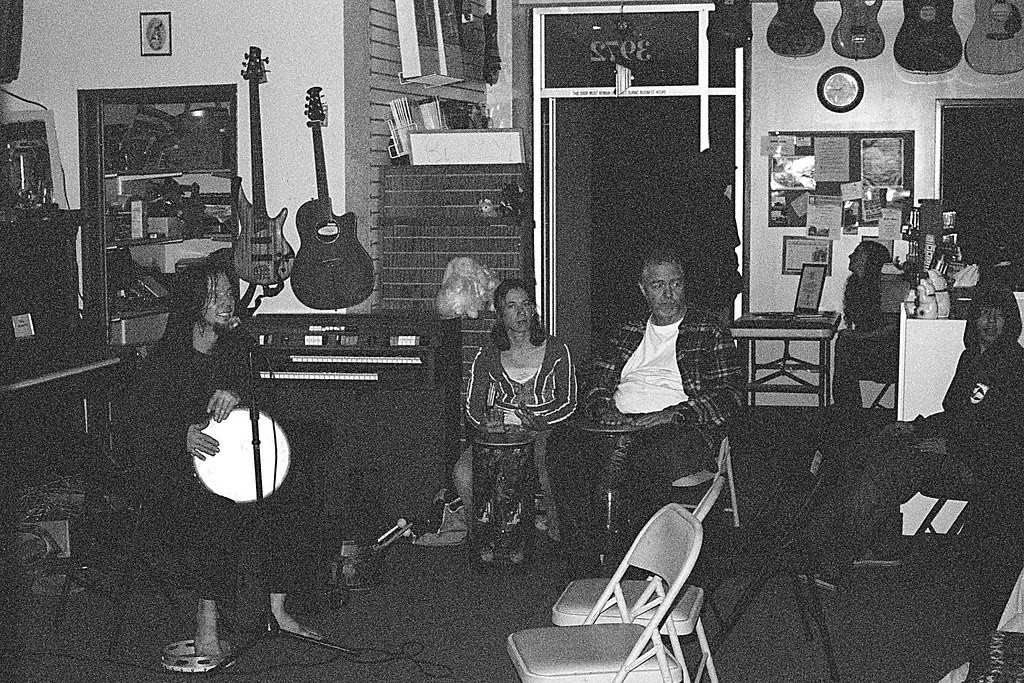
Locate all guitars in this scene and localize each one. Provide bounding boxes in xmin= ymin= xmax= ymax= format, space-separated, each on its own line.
xmin=229 ymin=42 xmax=296 ymax=285
xmin=706 ymin=2 xmax=753 ymax=47
xmin=290 ymin=86 xmax=376 ymax=310
xmin=892 ymin=1 xmax=961 ymax=74
xmin=965 ymin=2 xmax=1023 ymax=74
xmin=831 ymin=1 xmax=885 ymax=59
xmin=766 ymin=1 xmax=825 ymax=59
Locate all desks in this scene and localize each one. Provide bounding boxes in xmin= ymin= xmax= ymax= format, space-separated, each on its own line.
xmin=733 ymin=312 xmax=839 ymax=413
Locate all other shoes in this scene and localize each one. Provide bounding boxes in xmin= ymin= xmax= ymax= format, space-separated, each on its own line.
xmin=509 ymin=542 xmax=525 ymax=563
xmin=854 ymin=545 xmax=901 ymax=566
xmin=797 ymin=573 xmax=838 ymax=592
xmin=480 ymin=539 xmax=496 ymax=562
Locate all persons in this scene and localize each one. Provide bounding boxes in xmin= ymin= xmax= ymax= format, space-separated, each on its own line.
xmin=663 ymin=148 xmax=743 ymax=304
xmin=792 ymin=280 xmax=1024 ymax=590
xmin=453 ymin=279 xmax=578 ymax=543
xmin=830 ymin=240 xmax=908 ymax=416
xmin=579 ymin=248 xmax=747 ymax=579
xmin=128 ymin=262 xmax=324 ymax=665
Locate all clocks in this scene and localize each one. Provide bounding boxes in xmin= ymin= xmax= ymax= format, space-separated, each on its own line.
xmin=817 ymin=67 xmax=864 ymax=113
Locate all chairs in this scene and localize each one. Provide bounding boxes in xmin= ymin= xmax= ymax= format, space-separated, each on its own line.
xmin=670 ymin=435 xmax=740 ymax=528
xmin=50 ymin=427 xmax=176 ymax=651
xmin=552 ymin=475 xmax=728 ymax=683
xmin=505 ymin=501 xmax=705 ymax=683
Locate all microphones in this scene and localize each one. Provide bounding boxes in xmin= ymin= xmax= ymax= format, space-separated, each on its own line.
xmin=227 ymin=315 xmax=263 ymax=357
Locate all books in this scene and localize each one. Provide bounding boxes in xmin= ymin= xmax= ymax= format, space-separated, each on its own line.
xmin=389 ymin=96 xmax=448 ymax=154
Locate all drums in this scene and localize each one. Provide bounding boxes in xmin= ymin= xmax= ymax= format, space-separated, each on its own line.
xmin=191 ymin=406 xmax=293 ymax=505
xmin=577 ymin=418 xmax=644 ymax=581
xmin=471 ymin=428 xmax=538 ymax=576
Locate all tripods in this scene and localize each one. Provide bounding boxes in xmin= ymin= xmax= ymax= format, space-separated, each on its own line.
xmin=205 ymin=353 xmax=361 ymax=681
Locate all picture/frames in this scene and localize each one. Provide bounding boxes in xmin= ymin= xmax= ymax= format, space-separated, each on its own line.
xmin=794 ymin=264 xmax=825 ymax=311
xmin=140 ymin=11 xmax=172 ymax=57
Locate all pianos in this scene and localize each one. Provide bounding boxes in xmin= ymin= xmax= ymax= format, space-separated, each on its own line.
xmin=247 ymin=312 xmax=464 ymax=498
xmin=1 ymin=210 xmax=122 ymax=450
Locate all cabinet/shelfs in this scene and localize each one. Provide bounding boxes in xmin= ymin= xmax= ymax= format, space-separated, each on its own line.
xmin=77 ymin=85 xmax=238 ymax=442
